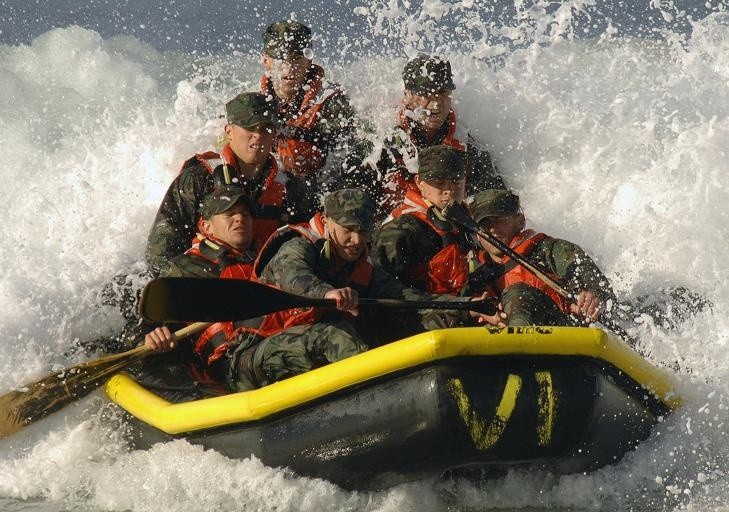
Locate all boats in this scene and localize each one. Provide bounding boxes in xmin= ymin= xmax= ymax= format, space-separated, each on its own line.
xmin=102 ymin=327 xmax=684 ymax=489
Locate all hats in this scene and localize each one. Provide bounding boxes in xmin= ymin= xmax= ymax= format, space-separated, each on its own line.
xmin=226 ymin=93 xmax=278 ymax=128
xmin=261 ymin=21 xmax=313 ymax=61
xmin=402 ymin=56 xmax=456 ymax=95
xmin=203 ymin=187 xmax=253 ymax=216
xmin=418 ymin=145 xmax=465 ymax=180
xmin=469 ymin=189 xmax=520 ymax=223
xmin=324 ymin=189 xmax=375 ymax=229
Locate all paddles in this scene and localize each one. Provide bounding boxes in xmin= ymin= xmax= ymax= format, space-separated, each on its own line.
xmin=0 ymin=321 xmax=215 ymax=440
xmin=139 ymin=277 xmax=499 ymax=324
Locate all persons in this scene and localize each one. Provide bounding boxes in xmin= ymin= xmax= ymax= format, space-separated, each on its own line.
xmin=95 ymin=189 xmax=262 ymax=404
xmin=223 ymin=188 xmax=508 ymax=405
xmin=145 ymin=90 xmax=315 ymax=276
xmin=376 ymin=56 xmax=519 ymax=214
xmin=465 ymin=187 xmax=621 ymax=331
xmin=370 ymin=146 xmax=477 ymax=348
xmin=257 ymin=20 xmax=357 ymax=190
